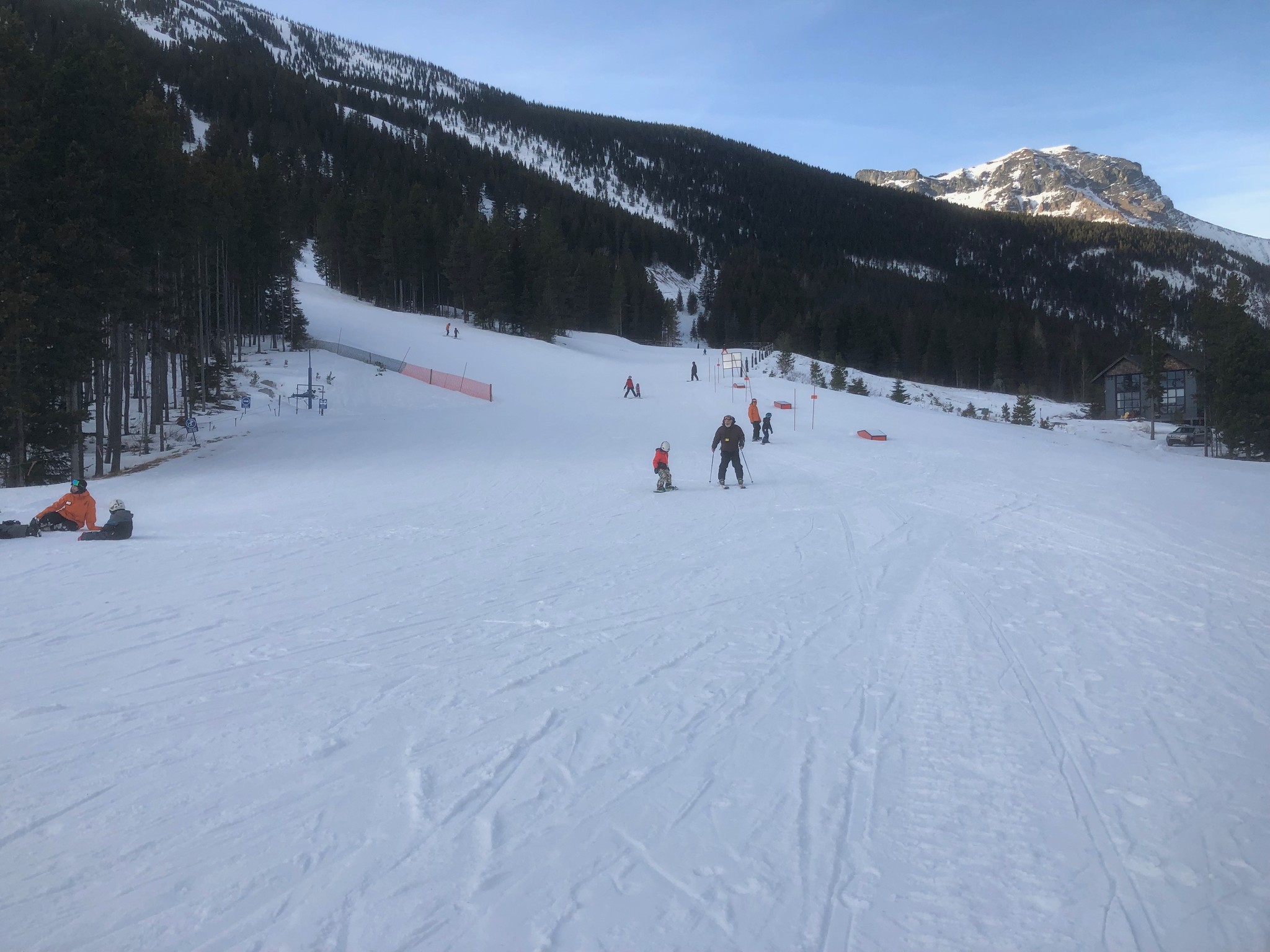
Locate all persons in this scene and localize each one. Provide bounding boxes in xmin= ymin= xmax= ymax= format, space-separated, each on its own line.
xmin=624 ymin=343 xmax=728 ymax=399
xmin=0 ymin=480 xmax=134 ymax=541
xmin=748 ymin=398 xmax=762 ymax=441
xmin=762 ymin=413 xmax=773 ymax=443
xmin=454 ymin=328 xmax=459 ymax=338
xmin=653 ymin=441 xmax=675 ymax=492
xmin=446 ymin=323 xmax=451 ymax=335
xmin=711 ymin=415 xmax=745 ymax=485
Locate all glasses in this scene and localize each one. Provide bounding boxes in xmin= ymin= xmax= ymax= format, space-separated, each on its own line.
xmin=724 ymin=416 xmax=732 ymax=420
xmin=71 ymin=480 xmax=80 ymax=486
xmin=753 ymin=400 xmax=757 ymax=402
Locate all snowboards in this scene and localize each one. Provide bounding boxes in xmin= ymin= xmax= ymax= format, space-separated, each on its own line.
xmin=443 ymin=335 xmax=454 ymax=337
xmin=453 ymin=338 xmax=460 ymax=339
xmin=760 ymin=442 xmax=770 ymax=445
xmin=687 ymin=380 xmax=702 ymax=382
xmin=653 ymin=487 xmax=678 ymax=493
xmin=632 ymin=398 xmax=643 ymax=399
xmin=752 ymin=439 xmax=763 ymax=441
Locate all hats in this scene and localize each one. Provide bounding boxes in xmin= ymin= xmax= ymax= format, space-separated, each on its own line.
xmin=72 ymin=479 xmax=87 ymax=489
xmin=752 ymin=399 xmax=757 ymax=403
xmin=722 ymin=415 xmax=736 ymax=424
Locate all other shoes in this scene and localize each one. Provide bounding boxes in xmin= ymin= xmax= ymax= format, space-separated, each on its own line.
xmin=665 ymin=483 xmax=676 ymax=489
xmin=77 ymin=532 xmax=86 ymax=541
xmin=753 ymin=437 xmax=762 ymax=441
xmin=738 ymin=478 xmax=743 ymax=485
xmin=657 ymin=487 xmax=666 ymax=492
xmin=719 ymin=478 xmax=725 ymax=485
xmin=762 ymin=441 xmax=770 ymax=443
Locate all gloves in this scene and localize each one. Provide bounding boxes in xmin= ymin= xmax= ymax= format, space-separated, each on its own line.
xmin=771 ymin=431 xmax=773 ymax=434
xmin=654 ymin=468 xmax=658 ymax=474
xmin=667 ymin=467 xmax=670 ymax=471
xmin=30 ymin=517 xmax=37 ymax=526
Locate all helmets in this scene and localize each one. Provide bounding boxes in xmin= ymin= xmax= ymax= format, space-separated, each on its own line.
xmin=661 ymin=441 xmax=670 ymax=451
xmin=628 ymin=376 xmax=632 ymax=379
xmin=766 ymin=413 xmax=772 ymax=418
xmin=108 ymin=499 xmax=125 ymax=511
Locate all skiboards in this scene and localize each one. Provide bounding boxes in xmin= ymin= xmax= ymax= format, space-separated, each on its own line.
xmin=717 ymin=482 xmax=746 ymax=489
